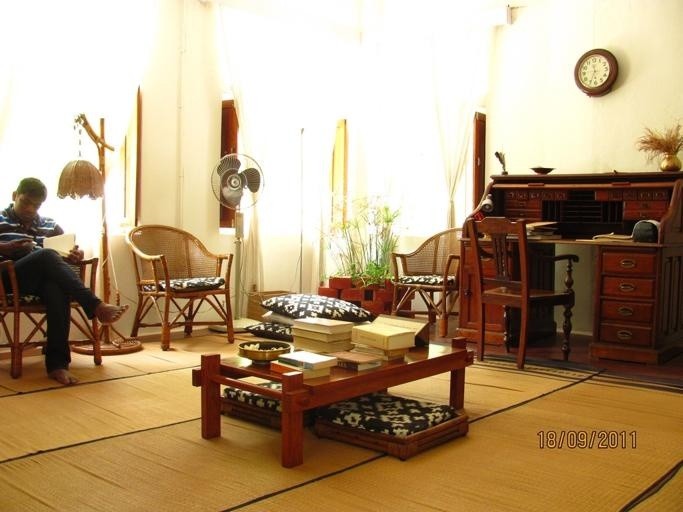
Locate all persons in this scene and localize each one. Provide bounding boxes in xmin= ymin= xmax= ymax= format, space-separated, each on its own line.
xmin=0 ymin=178 xmax=129 ymax=386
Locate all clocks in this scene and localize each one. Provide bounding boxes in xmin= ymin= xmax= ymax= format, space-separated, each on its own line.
xmin=571 ymin=48 xmax=619 ymax=98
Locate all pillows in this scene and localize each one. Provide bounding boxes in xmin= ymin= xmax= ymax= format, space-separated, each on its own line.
xmin=260 ymin=294 xmax=373 ymax=323
xmin=244 ymin=321 xmax=293 ymax=343
xmin=260 ymin=310 xmax=293 ymax=327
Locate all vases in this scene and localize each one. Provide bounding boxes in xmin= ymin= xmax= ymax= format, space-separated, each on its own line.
xmin=660 ymin=154 xmax=681 ymax=171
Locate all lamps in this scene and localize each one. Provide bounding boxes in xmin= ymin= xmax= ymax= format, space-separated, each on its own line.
xmin=56 ymin=113 xmax=142 ymax=356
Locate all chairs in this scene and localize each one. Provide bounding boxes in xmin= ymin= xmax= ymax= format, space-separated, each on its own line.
xmin=389 ymin=227 xmax=463 ymax=338
xmin=465 ymin=215 xmax=578 ymax=370
xmin=0 ymin=256 xmax=101 ymax=379
xmin=124 ymin=223 xmax=236 ymax=350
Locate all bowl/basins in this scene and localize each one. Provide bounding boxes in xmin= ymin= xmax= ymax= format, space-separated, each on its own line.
xmin=237 ymin=340 xmax=290 ymax=364
xmin=529 ymin=167 xmax=554 ymax=175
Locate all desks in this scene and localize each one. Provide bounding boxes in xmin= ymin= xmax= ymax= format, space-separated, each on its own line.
xmin=457 ymin=167 xmax=683 ymax=368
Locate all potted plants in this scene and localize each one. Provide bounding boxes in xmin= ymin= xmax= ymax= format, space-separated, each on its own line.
xmin=315 ymin=193 xmax=414 ymax=317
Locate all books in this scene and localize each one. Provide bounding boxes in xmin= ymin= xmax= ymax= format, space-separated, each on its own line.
xmin=34 ymin=233 xmax=76 ymax=259
xmin=483 ymin=222 xmax=633 ymax=242
xmin=270 ymin=317 xmax=416 ymax=379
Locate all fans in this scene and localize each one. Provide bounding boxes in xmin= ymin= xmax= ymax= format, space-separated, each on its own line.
xmin=208 ymin=152 xmax=266 ymax=332
